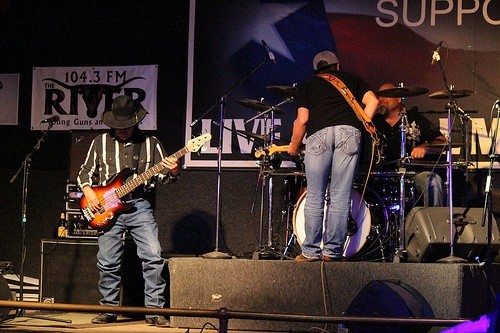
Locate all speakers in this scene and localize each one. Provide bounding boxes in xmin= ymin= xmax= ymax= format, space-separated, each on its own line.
xmin=0 ymin=273 xmax=14 ymax=317
xmin=69 ymin=132 xmax=101 ymax=182
xmin=40 ymin=239 xmax=145 ymax=308
xmin=343 ymin=278 xmax=436 ymax=333
xmin=402 ymin=205 xmax=500 ymax=263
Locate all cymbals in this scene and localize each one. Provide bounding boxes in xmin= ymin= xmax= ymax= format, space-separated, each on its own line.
xmin=427 ymin=90 xmax=476 ymax=99
xmin=239 ymin=130 xmax=283 ymax=147
xmin=378 ymin=88 xmax=430 ymax=98
xmin=237 ymin=98 xmax=289 ymax=116
xmin=267 ymin=86 xmax=300 ymax=96
xmin=426 ymin=142 xmax=465 ymax=148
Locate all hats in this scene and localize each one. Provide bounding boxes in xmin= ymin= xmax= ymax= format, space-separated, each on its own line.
xmin=101 ymin=95 xmax=147 ymax=130
xmin=313 ymin=51 xmax=339 ymax=70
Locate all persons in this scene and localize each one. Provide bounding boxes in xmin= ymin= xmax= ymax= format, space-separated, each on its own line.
xmin=76 ymin=96 xmax=180 ymax=328
xmin=362 ymin=84 xmax=445 ymax=207
xmin=289 ymin=52 xmax=379 ymax=262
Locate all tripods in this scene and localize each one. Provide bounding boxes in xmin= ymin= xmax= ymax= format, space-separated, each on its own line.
xmin=0 ymin=121 xmax=72 ymax=324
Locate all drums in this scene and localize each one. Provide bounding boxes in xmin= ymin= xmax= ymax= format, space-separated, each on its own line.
xmin=291 ymin=184 xmax=391 ymax=259
xmin=371 ymin=172 xmax=415 ymax=203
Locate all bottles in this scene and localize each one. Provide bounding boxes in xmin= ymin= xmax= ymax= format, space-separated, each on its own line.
xmin=338 ymin=312 xmax=345 ymax=333
xmin=57 ymin=212 xmax=65 ymax=239
xmin=15 ymin=293 xmax=22 ymax=316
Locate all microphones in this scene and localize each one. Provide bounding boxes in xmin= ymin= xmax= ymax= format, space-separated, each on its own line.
xmin=261 ymin=40 xmax=278 ymax=64
xmin=430 ymin=41 xmax=443 ymax=65
xmin=41 ymin=115 xmax=60 ymax=123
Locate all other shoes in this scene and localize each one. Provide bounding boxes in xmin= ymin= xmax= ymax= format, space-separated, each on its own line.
xmin=146 ymin=316 xmax=170 ymax=327
xmin=91 ymin=313 xmax=118 ymax=323
xmin=295 ymin=254 xmax=338 ymax=262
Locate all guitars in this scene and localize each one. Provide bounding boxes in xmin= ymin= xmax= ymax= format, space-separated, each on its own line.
xmin=79 ymin=131 xmax=212 ymax=231
xmin=253 ymin=121 xmax=382 ymax=162
